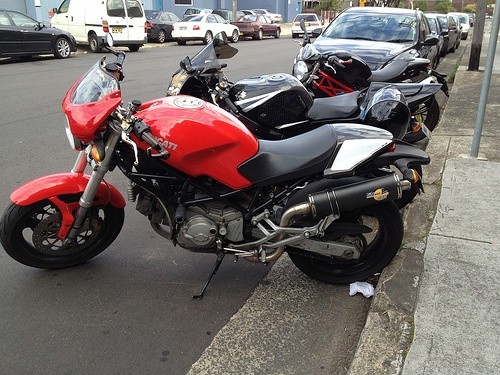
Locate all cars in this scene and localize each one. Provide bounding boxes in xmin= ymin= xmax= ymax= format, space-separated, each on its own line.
xmin=240 ymin=10 xmax=256 ymax=15
xmin=251 ymin=8 xmax=282 ymax=22
xmin=314 ymin=6 xmax=474 ymax=78
xmin=292 ymin=13 xmax=322 ymax=39
xmin=144 ymin=9 xmax=182 ymax=43
xmin=0 ymin=9 xmax=78 ymax=59
xmin=171 ymin=13 xmax=240 ymax=45
xmin=230 ymin=14 xmax=282 ymax=39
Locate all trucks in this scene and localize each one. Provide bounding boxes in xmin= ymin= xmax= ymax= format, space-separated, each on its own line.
xmin=49 ymin=0 xmax=148 ymax=53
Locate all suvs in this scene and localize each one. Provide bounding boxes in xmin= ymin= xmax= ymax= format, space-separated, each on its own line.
xmin=212 ymin=9 xmax=246 ymax=22
xmin=183 ymin=7 xmax=213 ymax=18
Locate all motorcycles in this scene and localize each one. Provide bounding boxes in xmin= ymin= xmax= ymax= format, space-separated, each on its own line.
xmin=0 ymin=29 xmax=431 ymax=300
xmin=291 ymin=18 xmax=450 ymax=142
xmin=166 ymin=32 xmax=444 ymax=210
